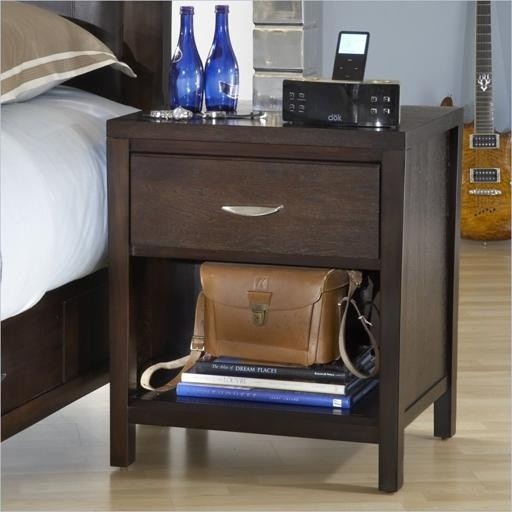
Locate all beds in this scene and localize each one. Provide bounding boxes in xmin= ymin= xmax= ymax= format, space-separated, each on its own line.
xmin=1 ymin=14 xmax=172 ymax=439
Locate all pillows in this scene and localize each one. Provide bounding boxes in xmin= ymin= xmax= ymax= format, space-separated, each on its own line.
xmin=1 ymin=0 xmax=138 ymax=104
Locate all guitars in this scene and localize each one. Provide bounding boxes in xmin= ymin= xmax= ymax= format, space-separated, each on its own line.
xmin=439 ymin=1 xmax=511 ymax=240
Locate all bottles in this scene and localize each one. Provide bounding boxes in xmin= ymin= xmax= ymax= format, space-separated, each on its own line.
xmin=167 ymin=6 xmax=205 ymax=113
xmin=203 ymin=4 xmax=241 ymax=109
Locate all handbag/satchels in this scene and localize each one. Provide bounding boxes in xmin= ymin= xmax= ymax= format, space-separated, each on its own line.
xmin=199 ymin=261 xmax=359 ymax=367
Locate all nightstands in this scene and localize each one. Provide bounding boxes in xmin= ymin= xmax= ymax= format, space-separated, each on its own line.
xmin=105 ymin=100 xmax=464 ymax=493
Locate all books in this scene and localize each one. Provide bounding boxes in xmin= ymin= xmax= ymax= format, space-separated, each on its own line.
xmin=175 ymin=338 xmax=379 ymax=410
